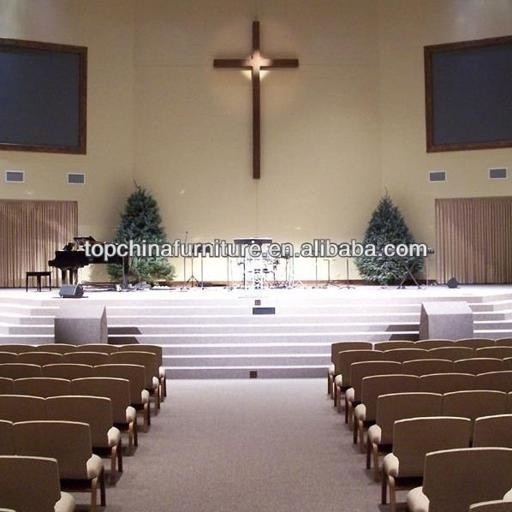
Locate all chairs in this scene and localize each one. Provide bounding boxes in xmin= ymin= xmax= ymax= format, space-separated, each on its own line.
xmin=0 ymin=341 xmax=168 ymax=512
xmin=325 ymin=332 xmax=512 ymax=511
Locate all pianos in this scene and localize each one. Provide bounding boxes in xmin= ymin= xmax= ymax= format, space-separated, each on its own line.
xmin=47 ymin=235 xmax=130 ymax=284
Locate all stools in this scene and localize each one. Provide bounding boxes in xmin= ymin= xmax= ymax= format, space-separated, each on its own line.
xmin=25 ymin=271 xmax=52 ymax=291
